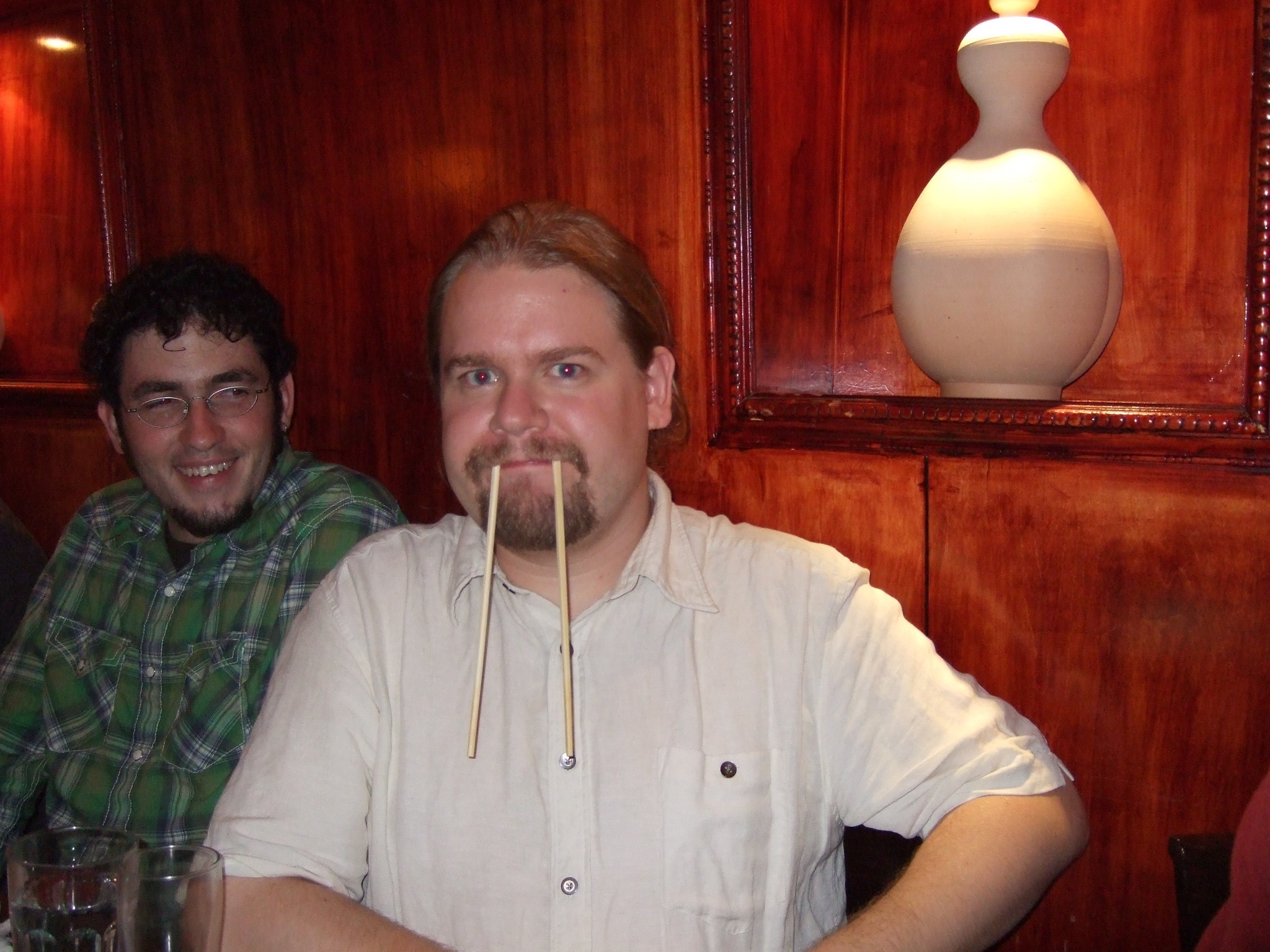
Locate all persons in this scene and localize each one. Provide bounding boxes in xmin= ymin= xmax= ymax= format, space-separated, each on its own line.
xmin=1194 ymin=769 xmax=1270 ymax=951
xmin=186 ymin=202 xmax=1089 ymax=951
xmin=0 ymin=250 xmax=410 ymax=952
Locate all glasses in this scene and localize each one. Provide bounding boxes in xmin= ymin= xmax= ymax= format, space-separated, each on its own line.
xmin=120 ymin=372 xmax=274 ymax=428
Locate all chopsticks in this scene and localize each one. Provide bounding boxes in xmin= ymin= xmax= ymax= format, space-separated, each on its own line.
xmin=466 ymin=458 xmax=576 ymax=761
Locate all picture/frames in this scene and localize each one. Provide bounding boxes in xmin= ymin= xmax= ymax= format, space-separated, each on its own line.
xmin=1 ymin=1 xmax=138 ymax=418
xmin=698 ymin=0 xmax=1270 ymax=472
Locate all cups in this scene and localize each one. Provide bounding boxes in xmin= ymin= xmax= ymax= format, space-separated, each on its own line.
xmin=114 ymin=842 xmax=226 ymax=952
xmin=7 ymin=825 xmax=141 ymax=952
